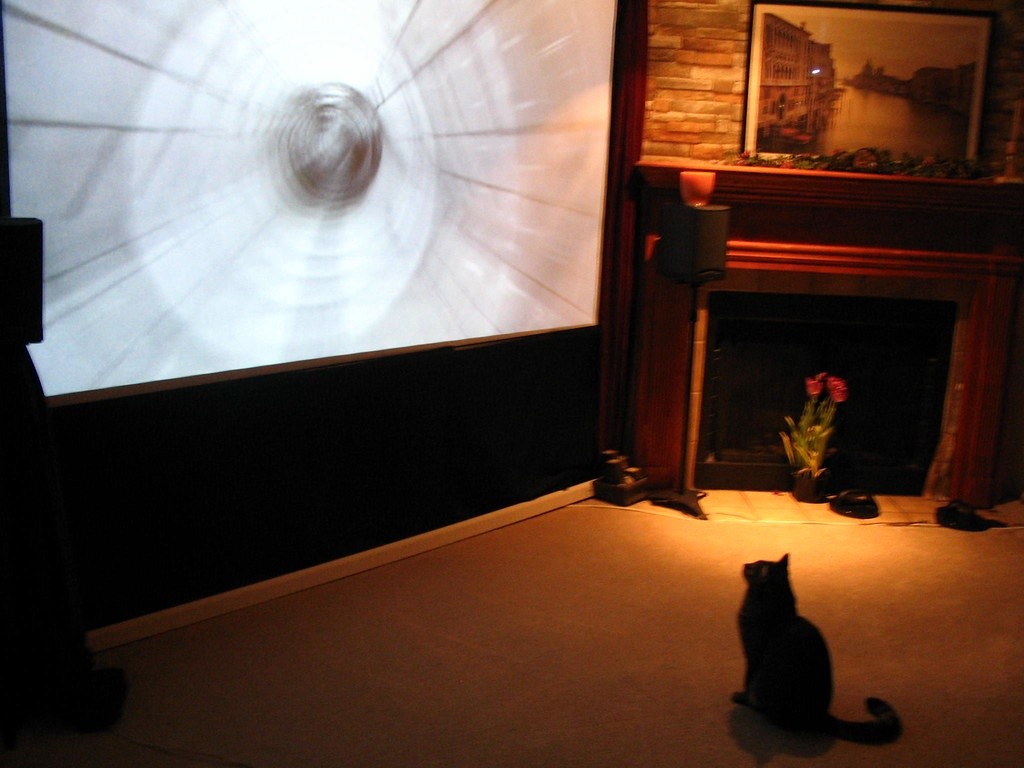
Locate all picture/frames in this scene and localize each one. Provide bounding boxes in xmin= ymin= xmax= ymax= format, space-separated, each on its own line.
xmin=739 ymin=0 xmax=998 ymax=178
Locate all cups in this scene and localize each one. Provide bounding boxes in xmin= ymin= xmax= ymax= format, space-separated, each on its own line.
xmin=680 ymin=171 xmax=716 ymax=207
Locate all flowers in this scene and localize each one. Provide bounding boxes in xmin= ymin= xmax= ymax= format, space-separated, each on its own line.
xmin=777 ymin=372 xmax=848 ymax=479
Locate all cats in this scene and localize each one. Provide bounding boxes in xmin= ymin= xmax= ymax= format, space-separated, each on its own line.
xmin=725 ymin=552 xmax=906 ymax=760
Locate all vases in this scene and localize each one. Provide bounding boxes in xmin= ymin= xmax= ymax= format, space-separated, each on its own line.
xmin=794 ymin=476 xmax=826 ymax=504
